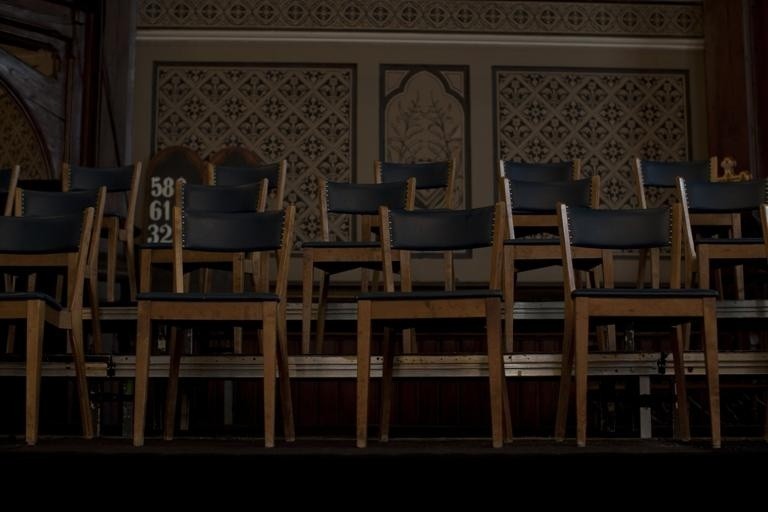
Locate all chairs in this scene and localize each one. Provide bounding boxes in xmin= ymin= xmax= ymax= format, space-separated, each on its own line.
xmin=131 ymin=203 xmax=298 ymax=449
xmin=146 ymin=159 xmax=765 ymax=357
xmin=552 ymin=200 xmax=727 ymax=450
xmin=353 ymin=199 xmax=513 ymax=449
xmin=2 ymin=158 xmax=144 ymax=450
